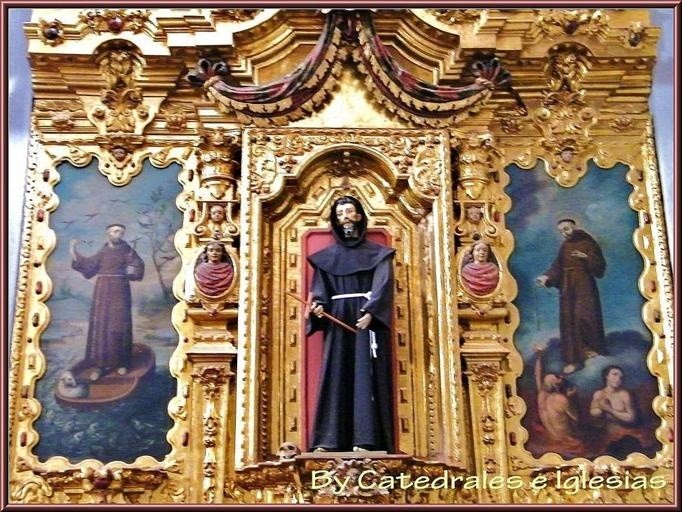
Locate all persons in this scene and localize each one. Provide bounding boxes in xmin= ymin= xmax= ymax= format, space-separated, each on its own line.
xmin=531 ymin=354 xmax=582 ymax=449
xmin=463 ymin=204 xmax=483 ymax=234
xmin=533 ymin=215 xmax=606 ymax=373
xmin=299 ymin=193 xmax=399 ymax=455
xmin=460 ymin=242 xmax=499 ymax=297
xmin=588 ymin=364 xmax=641 ymax=456
xmin=195 ymin=243 xmax=231 ymax=298
xmin=68 ymin=221 xmax=146 ymax=385
xmin=204 ymin=205 xmax=226 ymax=237
